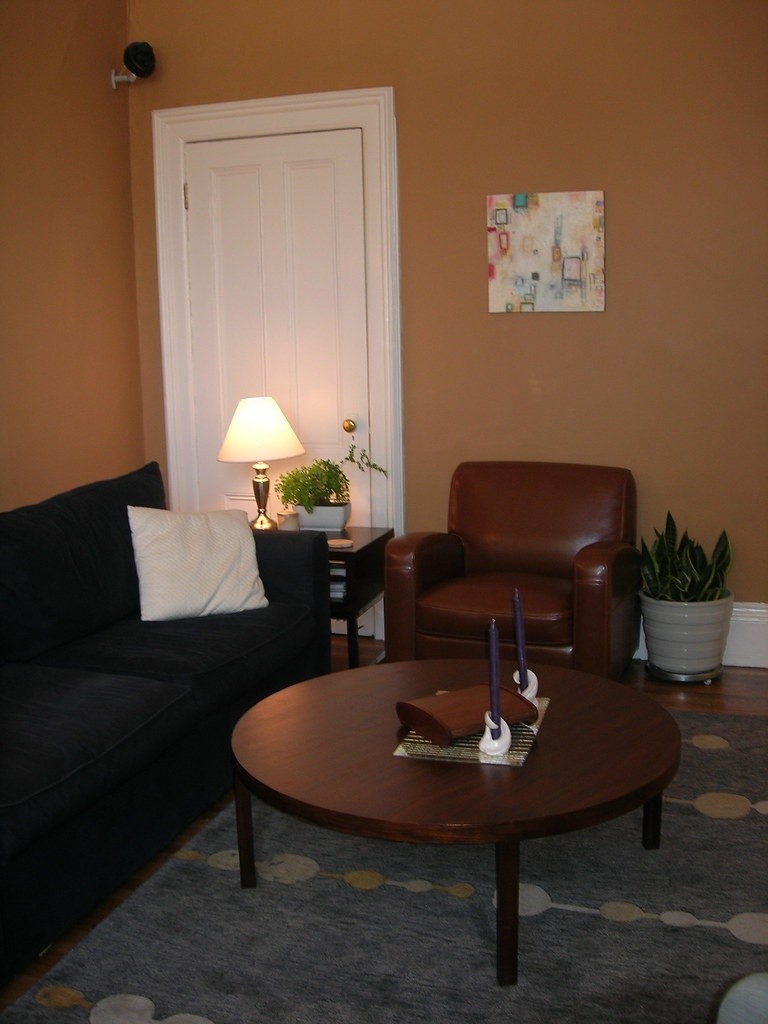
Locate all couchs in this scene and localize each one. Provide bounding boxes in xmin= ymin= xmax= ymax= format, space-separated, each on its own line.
xmin=0 ymin=463 xmax=333 ymax=977
xmin=387 ymin=459 xmax=639 ymax=677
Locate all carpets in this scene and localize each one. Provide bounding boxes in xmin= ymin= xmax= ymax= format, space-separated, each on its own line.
xmin=0 ymin=700 xmax=768 ymax=1024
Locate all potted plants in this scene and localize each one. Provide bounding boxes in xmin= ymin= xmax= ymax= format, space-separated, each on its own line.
xmin=636 ymin=511 xmax=736 ymax=685
xmin=276 ymin=443 xmax=391 ymax=533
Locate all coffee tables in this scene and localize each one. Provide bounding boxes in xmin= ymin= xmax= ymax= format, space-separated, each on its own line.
xmin=231 ymin=658 xmax=683 ymax=984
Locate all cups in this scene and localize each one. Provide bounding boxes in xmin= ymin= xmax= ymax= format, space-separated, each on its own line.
xmin=276 ymin=511 xmax=300 ymax=531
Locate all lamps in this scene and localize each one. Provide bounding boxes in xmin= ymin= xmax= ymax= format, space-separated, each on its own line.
xmin=219 ymin=395 xmax=307 ymax=529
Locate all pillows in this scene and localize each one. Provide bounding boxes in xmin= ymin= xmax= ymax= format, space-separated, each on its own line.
xmin=125 ymin=506 xmax=269 ymax=623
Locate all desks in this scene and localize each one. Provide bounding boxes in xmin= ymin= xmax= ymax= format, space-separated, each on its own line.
xmin=304 ymin=527 xmax=395 ymax=669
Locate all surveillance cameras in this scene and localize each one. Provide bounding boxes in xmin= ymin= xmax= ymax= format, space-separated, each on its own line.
xmin=124 ymin=42 xmax=155 ymax=78
